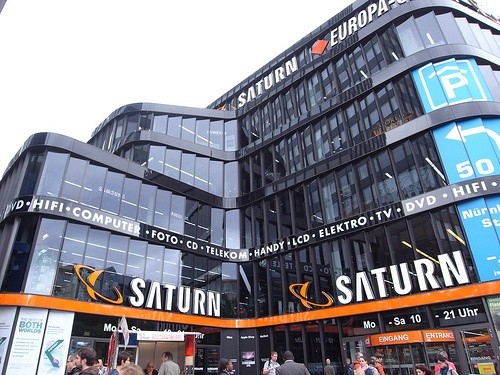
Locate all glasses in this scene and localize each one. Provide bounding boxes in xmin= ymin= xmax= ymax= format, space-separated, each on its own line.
xmin=416 ymin=371 xmax=423 ymax=373
xmin=359 ymin=360 xmax=365 ymax=362
xmin=436 ymin=362 xmax=443 ymax=366
xmin=148 ymin=365 xmax=154 ymax=369
xmin=66 ymin=360 xmax=73 ymax=364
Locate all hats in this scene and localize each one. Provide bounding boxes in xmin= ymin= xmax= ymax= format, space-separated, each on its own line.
xmin=375 ymin=352 xmax=384 ymax=357
xmin=282 ymin=350 xmax=294 ymax=359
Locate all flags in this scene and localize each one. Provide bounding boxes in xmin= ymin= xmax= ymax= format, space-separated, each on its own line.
xmin=118 ymin=315 xmax=129 ymax=349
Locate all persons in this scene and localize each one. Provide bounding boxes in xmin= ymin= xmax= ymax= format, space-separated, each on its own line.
xmin=415 ymin=351 xmax=459 ymax=375
xmin=218 ymin=358 xmax=233 ymax=375
xmin=275 ymin=350 xmax=311 ymax=375
xmin=344 ymin=352 xmax=385 ymax=375
xmin=324 ymin=359 xmax=335 ymax=375
xmin=262 ymin=351 xmax=280 ymax=375
xmin=66 ymin=347 xmax=180 ymax=375
